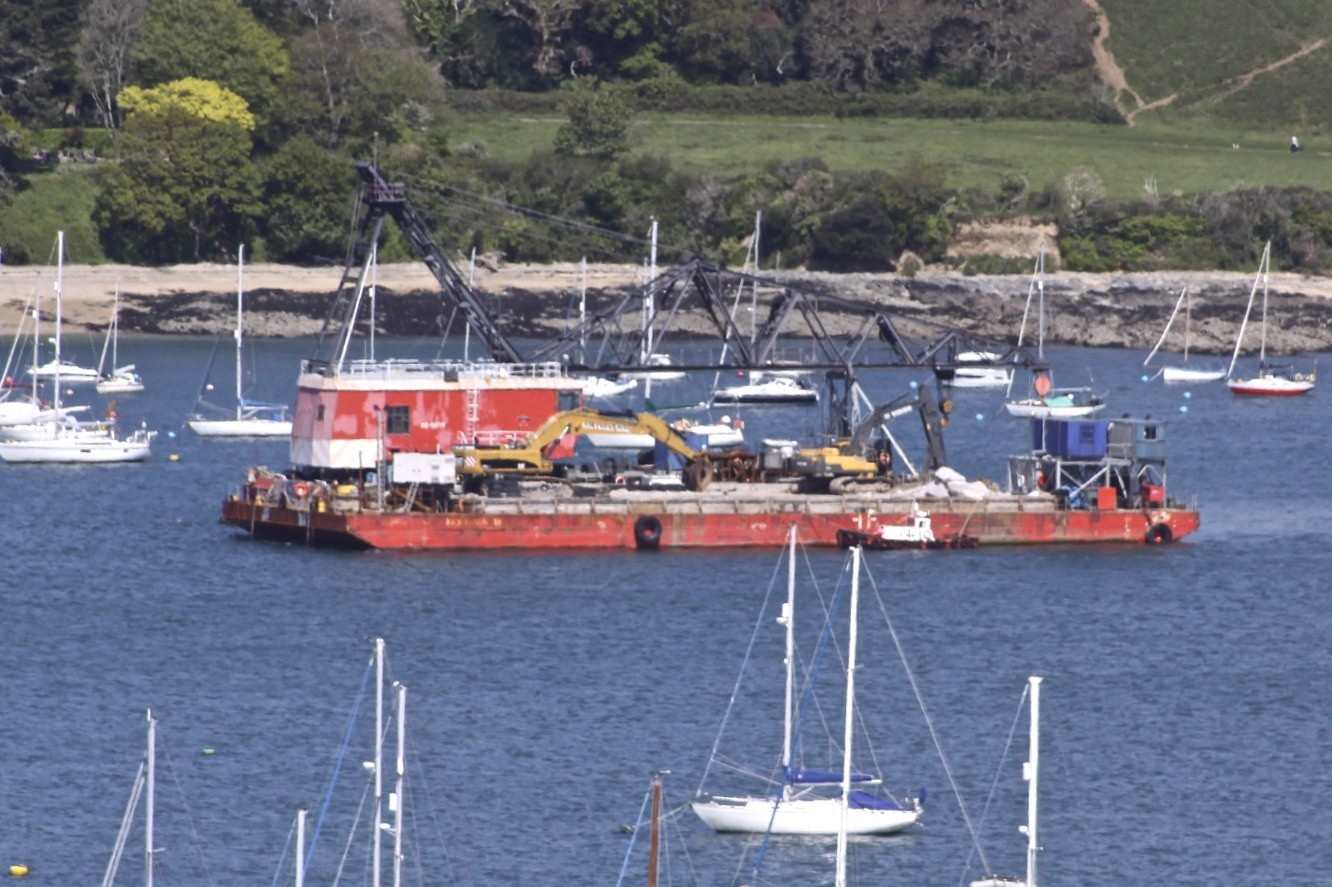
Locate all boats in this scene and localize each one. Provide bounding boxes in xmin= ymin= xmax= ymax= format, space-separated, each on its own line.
xmin=940 ymin=349 xmax=1009 ymax=389
xmin=668 ymin=416 xmax=746 ymax=452
xmin=216 ymin=152 xmax=1207 ymax=560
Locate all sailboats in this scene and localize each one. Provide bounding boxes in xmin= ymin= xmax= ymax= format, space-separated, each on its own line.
xmin=688 ymin=525 xmax=924 ymax=837
xmin=0 ymin=230 xmax=161 ymax=465
xmin=94 ymin=287 xmax=149 ymax=396
xmin=1219 ymin=238 xmax=1319 ymax=397
xmin=1004 ymin=253 xmax=1107 ymax=421
xmin=188 ymin=245 xmax=297 ymax=439
xmin=709 ymin=212 xmax=823 ymax=406
xmin=614 ymin=219 xmax=686 ymax=381
xmin=1143 ymin=287 xmax=1228 ymax=387
xmin=560 ymin=256 xmax=639 ymax=398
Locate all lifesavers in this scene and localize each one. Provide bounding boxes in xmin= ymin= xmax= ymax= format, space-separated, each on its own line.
xmin=635 ymin=516 xmax=661 ymax=545
xmin=1145 ymin=522 xmax=1172 ymax=545
